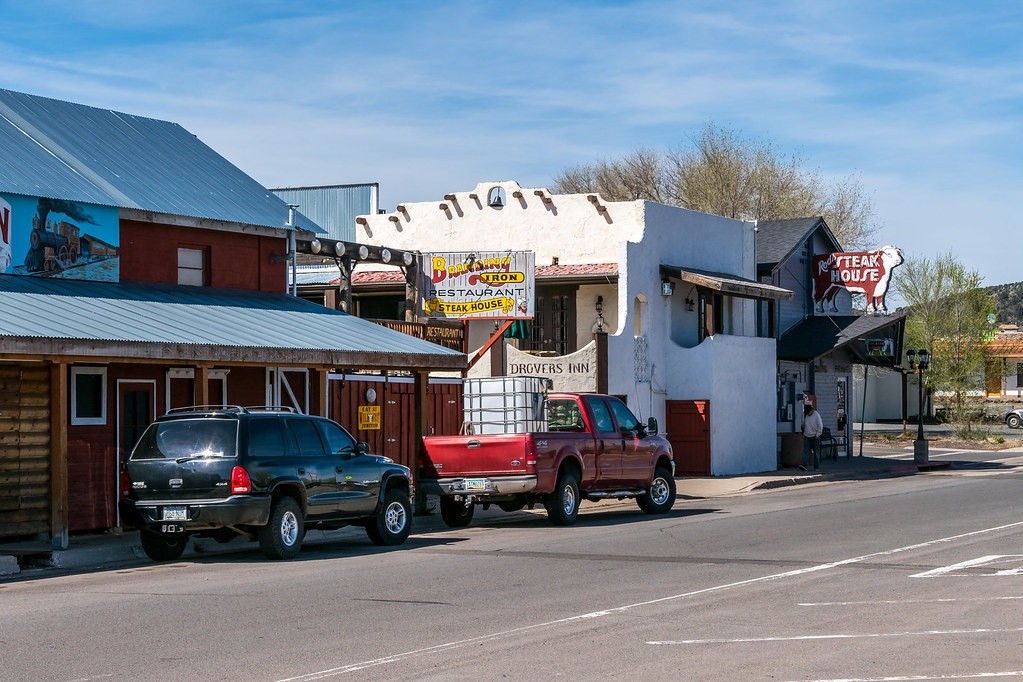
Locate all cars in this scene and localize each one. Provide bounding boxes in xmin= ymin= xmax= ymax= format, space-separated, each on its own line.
xmin=999 ymin=407 xmax=1023 ymax=429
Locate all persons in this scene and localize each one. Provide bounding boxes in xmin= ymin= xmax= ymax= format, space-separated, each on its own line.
xmin=799 ymin=405 xmax=823 ymax=471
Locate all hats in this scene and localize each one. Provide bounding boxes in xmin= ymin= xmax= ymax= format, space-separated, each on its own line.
xmin=803 ymin=405 xmax=811 ymax=414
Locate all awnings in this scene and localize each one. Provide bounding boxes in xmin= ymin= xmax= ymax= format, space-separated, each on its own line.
xmin=288 ymin=272 xmax=340 ymax=290
xmin=534 ymin=262 xmax=619 ymax=280
xmin=0 ymin=274 xmax=469 ymax=370
xmin=659 ymin=264 xmax=795 ymax=302
xmin=777 ymin=314 xmax=906 ymax=365
xmin=330 ymin=270 xmax=406 ymax=289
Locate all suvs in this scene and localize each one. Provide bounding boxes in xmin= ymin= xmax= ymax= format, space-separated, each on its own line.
xmin=121 ymin=404 xmax=416 ymax=560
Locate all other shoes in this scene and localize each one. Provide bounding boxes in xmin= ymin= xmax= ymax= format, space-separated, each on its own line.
xmin=814 ymin=466 xmax=819 ymax=471
xmin=799 ymin=464 xmax=808 ymax=471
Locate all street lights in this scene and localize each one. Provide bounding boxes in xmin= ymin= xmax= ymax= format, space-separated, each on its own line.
xmin=906 ymin=348 xmax=932 ymax=463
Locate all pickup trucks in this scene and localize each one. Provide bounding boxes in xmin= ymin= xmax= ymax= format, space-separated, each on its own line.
xmin=414 ymin=392 xmax=679 ymax=526
